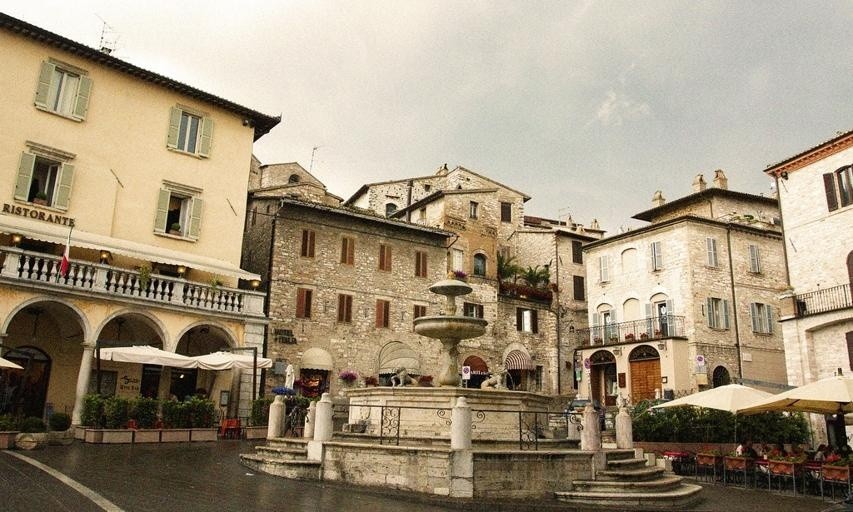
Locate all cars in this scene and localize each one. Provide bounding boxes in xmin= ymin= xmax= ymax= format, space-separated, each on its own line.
xmin=632 ymin=397 xmax=674 ymax=417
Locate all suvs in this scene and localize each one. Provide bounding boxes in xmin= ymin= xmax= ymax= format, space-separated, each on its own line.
xmin=565 ymin=399 xmax=606 ymax=430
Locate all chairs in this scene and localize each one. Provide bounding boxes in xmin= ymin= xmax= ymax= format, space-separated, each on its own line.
xmin=220 ymin=416 xmax=249 ymax=440
xmin=658 ymin=448 xmax=852 ymax=503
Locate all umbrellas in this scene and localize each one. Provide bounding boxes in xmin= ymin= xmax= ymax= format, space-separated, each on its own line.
xmin=730 ymin=366 xmax=852 ymax=415
xmin=91 ymin=342 xmax=198 ymax=372
xmin=644 ymin=382 xmax=786 ymax=455
xmin=0 ymin=355 xmax=24 ymax=371
xmin=187 ymin=349 xmax=273 ymax=372
xmin=279 ymin=363 xmax=295 ymax=397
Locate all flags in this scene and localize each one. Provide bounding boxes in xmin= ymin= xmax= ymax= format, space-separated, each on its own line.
xmin=60 ymin=237 xmax=70 ymax=277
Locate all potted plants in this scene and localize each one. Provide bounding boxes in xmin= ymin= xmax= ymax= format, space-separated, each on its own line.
xmin=0 ymin=411 xmax=77 ymax=451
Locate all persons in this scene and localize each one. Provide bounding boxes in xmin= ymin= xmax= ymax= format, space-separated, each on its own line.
xmin=823 ymin=442 xmax=835 ymax=459
xmin=835 ymin=433 xmax=853 ymax=457
xmin=389 ymin=364 xmax=415 ymax=387
xmin=183 ymin=392 xmax=193 ymax=401
xmin=168 ymin=392 xmax=178 ymax=401
xmin=758 ymin=439 xmax=771 ymax=452
xmin=810 ymin=444 xmax=826 ymax=478
xmin=770 ymin=441 xmax=789 ymax=459
xmin=733 ymin=435 xmax=758 ymax=459
xmin=480 ymin=367 xmax=507 ymax=391
